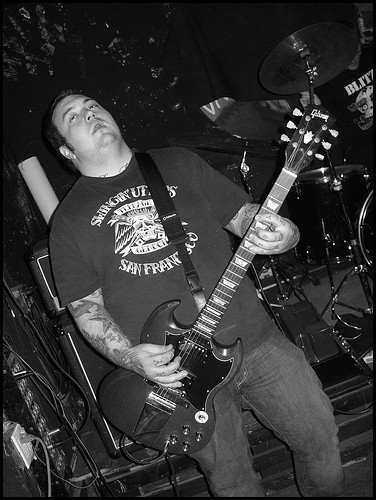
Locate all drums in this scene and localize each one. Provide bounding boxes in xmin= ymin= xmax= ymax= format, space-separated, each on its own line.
xmin=357 ymin=193 xmax=376 ymax=278
xmin=289 ymin=160 xmax=371 ymax=221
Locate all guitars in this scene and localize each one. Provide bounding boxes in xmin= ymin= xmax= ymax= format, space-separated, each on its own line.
xmin=95 ymin=100 xmax=346 ymax=457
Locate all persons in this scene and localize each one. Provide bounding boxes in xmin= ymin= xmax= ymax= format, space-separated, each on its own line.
xmin=315 ymin=43 xmax=376 ymax=185
xmin=44 ymin=91 xmax=346 ymax=500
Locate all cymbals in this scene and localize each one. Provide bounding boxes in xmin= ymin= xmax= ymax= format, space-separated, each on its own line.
xmin=258 ymin=21 xmax=359 ymax=95
